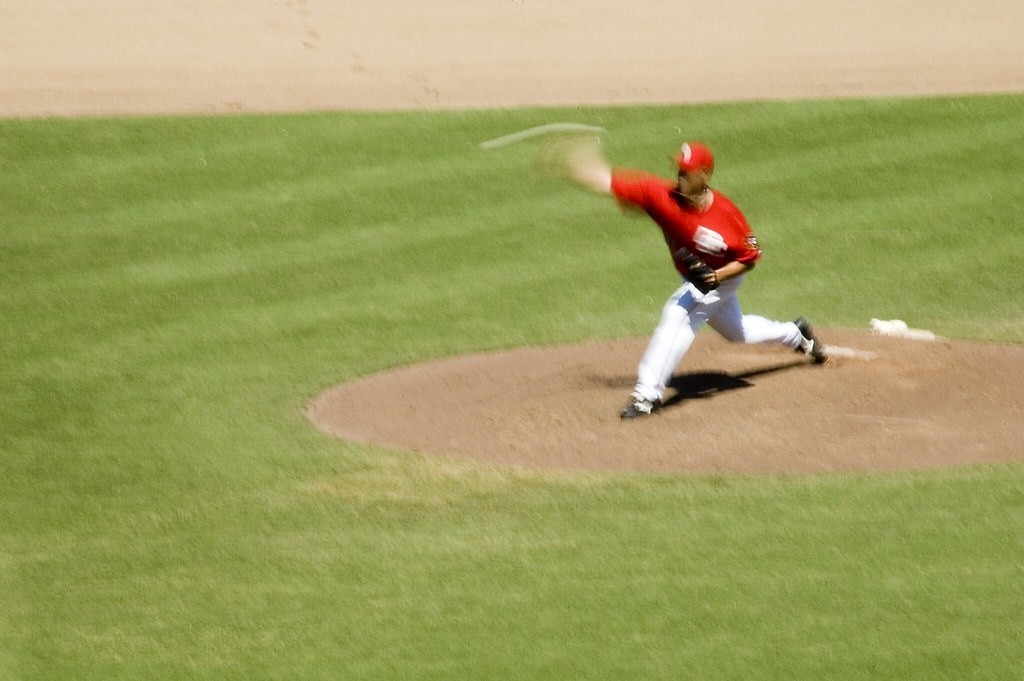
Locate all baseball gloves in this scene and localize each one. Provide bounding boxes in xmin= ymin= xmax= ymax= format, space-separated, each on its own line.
xmin=673 ymin=246 xmax=720 ymax=295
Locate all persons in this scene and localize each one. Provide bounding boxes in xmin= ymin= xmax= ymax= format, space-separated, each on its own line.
xmin=559 ymin=139 xmax=831 ymax=418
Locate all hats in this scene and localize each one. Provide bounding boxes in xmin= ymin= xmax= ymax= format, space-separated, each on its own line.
xmin=675 ymin=141 xmax=714 ymax=172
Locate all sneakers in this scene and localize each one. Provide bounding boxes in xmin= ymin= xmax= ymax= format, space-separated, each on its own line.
xmin=794 ymin=317 xmax=828 ymax=364
xmin=620 ymin=392 xmax=662 ymax=420
